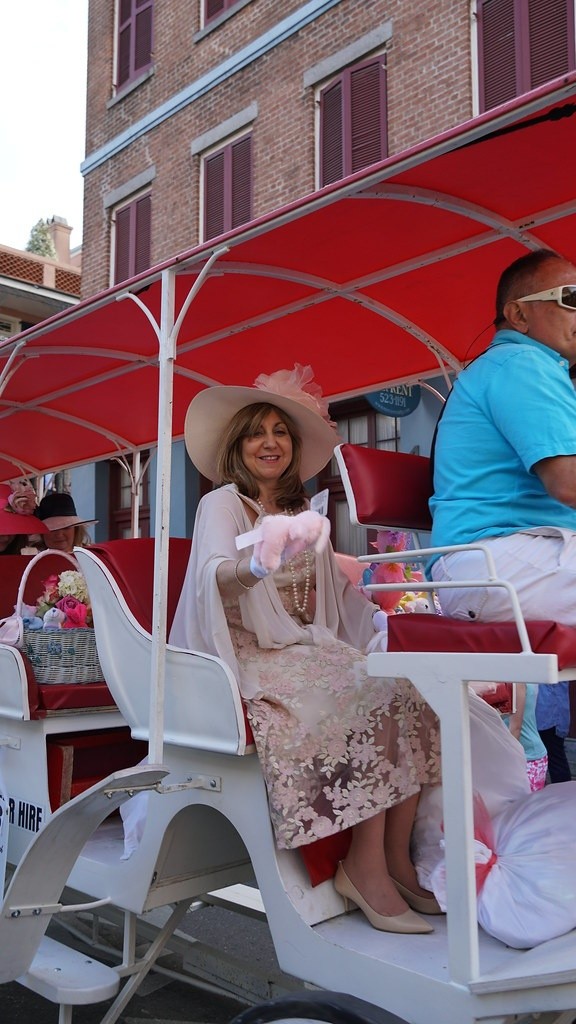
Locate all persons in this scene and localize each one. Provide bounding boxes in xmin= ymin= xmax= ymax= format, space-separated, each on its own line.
xmin=167 ymin=363 xmax=448 ymax=935
xmin=0 ymin=483 xmax=92 ymax=554
xmin=534 ymin=680 xmax=572 ymax=784
xmin=421 ymin=248 xmax=575 ymax=625
xmin=501 ymin=683 xmax=547 ymax=793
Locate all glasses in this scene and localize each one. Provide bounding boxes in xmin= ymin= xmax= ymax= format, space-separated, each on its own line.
xmin=514 ymin=285 xmax=576 ymax=309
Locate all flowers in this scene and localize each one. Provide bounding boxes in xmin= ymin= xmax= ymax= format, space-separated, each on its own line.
xmin=36 ymin=570 xmax=89 ymax=629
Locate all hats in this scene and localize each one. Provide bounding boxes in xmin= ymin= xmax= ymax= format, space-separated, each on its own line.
xmin=0 ymin=484 xmax=50 ymax=534
xmin=184 ymin=362 xmax=343 ymax=484
xmin=34 ymin=494 xmax=99 ymax=532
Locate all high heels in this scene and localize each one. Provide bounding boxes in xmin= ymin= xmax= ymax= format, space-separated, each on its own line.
xmin=334 ymin=859 xmax=446 ymax=934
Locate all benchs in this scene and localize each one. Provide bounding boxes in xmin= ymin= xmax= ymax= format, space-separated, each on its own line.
xmin=334 ymin=442 xmax=575 ymax=980
xmin=75 ymin=536 xmax=428 ymax=926
xmin=0 ymin=555 xmax=142 ymax=830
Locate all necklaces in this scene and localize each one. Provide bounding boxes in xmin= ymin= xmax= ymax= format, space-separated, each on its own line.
xmin=254 ymin=493 xmax=311 ymax=612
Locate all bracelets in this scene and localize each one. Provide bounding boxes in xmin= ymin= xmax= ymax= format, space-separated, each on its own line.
xmin=235 ymin=558 xmax=255 ymax=590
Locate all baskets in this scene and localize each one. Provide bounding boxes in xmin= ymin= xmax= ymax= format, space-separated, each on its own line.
xmin=13 ymin=548 xmax=105 ymax=684
